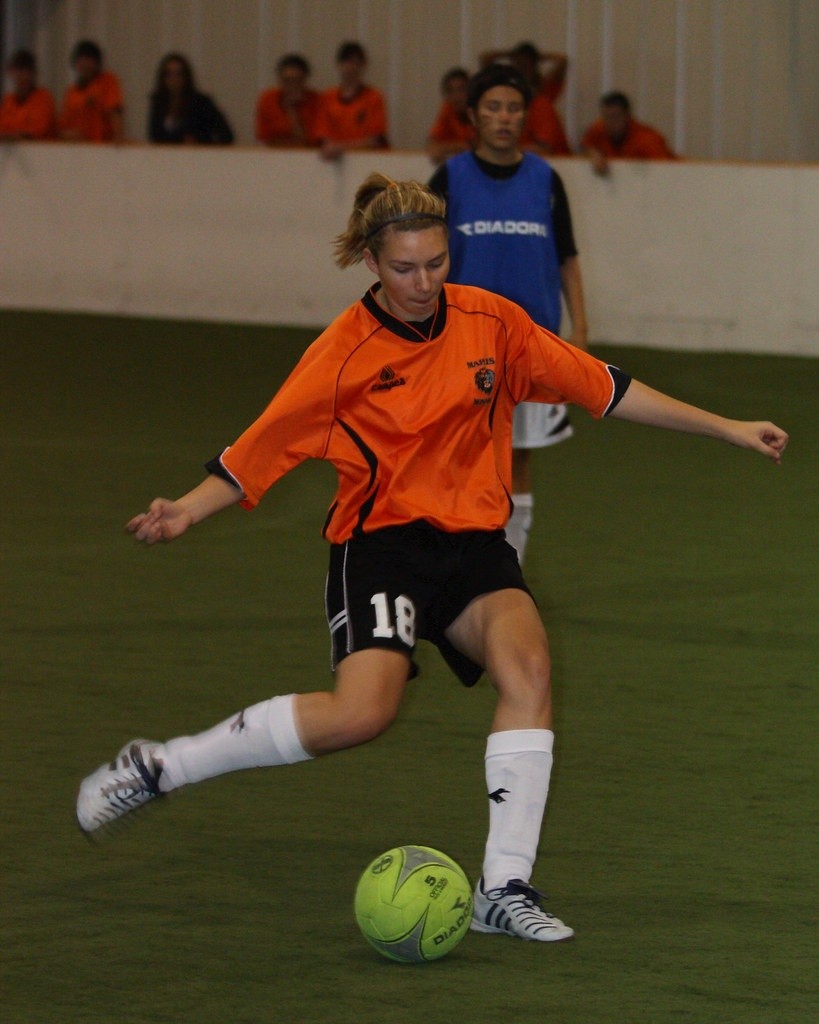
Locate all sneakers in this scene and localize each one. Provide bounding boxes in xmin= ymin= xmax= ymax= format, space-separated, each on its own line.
xmin=467 ymin=876 xmax=576 ymax=942
xmin=76 ymin=737 xmax=162 ymax=832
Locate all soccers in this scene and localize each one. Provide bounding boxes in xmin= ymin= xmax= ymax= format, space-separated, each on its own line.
xmin=353 ymin=843 xmax=475 ymax=966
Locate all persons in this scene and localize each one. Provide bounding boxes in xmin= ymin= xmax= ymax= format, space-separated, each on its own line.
xmin=76 ymin=175 xmax=787 ymax=943
xmin=429 ymin=43 xmax=571 ymax=159
xmin=255 ymin=39 xmax=389 ymax=157
xmin=60 ymin=41 xmax=124 ymax=145
xmin=0 ymin=51 xmax=54 ymax=139
xmin=146 ymin=54 xmax=234 ymax=145
xmin=424 ymin=65 xmax=589 ymax=565
xmin=581 ymin=91 xmax=670 ymax=176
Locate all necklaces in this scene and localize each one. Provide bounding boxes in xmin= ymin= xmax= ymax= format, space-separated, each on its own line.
xmin=370 ymin=281 xmax=438 ymax=342
